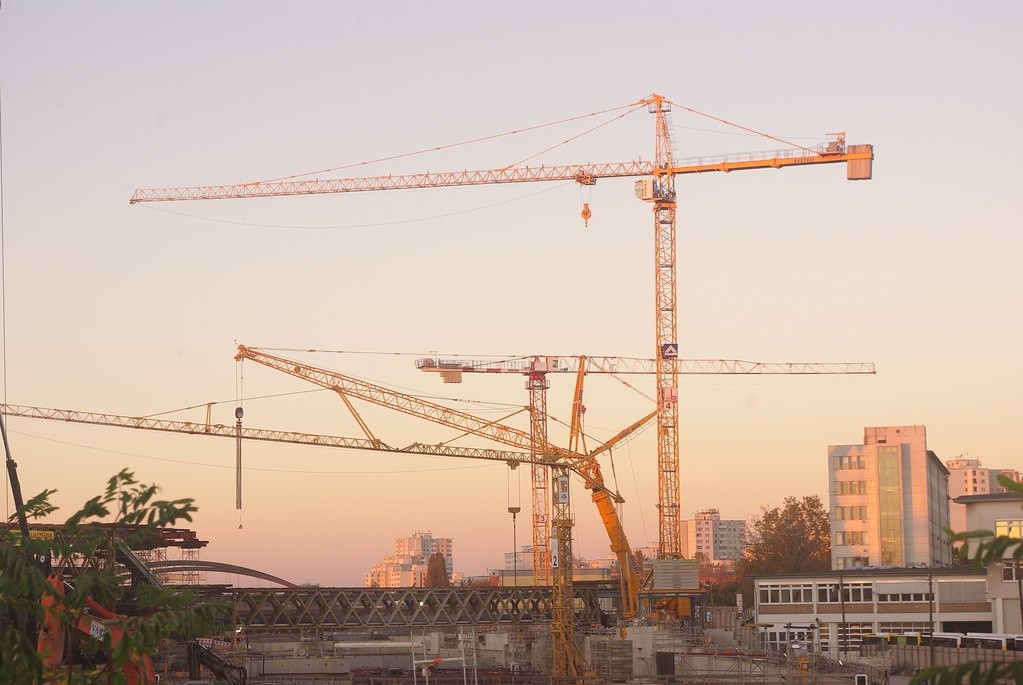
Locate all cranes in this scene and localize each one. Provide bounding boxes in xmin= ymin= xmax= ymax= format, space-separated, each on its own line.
xmin=0 ymin=91 xmax=874 ymax=685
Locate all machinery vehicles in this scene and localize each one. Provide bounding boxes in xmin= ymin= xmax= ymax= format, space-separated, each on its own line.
xmin=0 ymin=412 xmax=246 ymax=685
